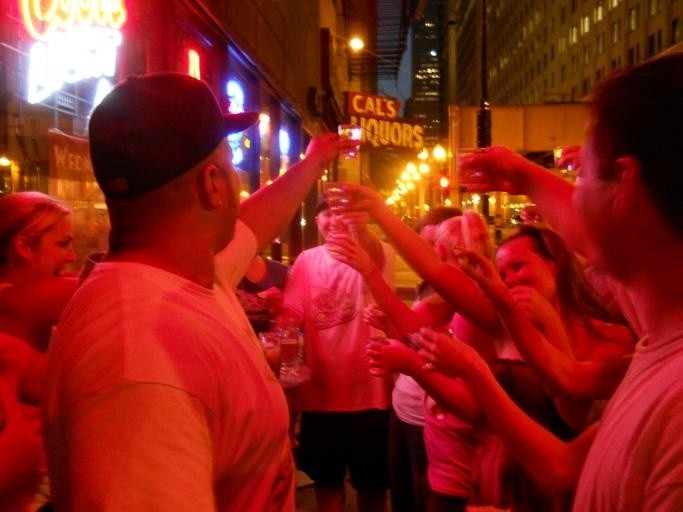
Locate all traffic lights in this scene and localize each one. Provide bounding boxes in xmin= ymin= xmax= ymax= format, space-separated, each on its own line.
xmin=439 ymin=172 xmax=450 ymax=197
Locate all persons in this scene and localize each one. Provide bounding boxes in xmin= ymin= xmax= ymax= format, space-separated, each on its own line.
xmin=270 ymin=53 xmax=683 ymax=511
xmin=1 ymin=74 xmax=363 ymax=512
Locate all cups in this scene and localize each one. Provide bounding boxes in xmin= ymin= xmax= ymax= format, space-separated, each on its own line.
xmin=259 ymin=325 xmax=300 ymax=380
xmin=456 ymin=147 xmax=489 ymax=187
xmin=338 ymin=124 xmax=362 ymax=159
xmin=553 ymin=145 xmax=577 ymax=177
xmin=327 ymin=181 xmax=349 ymax=210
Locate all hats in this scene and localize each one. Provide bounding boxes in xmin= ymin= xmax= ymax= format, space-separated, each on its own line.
xmin=89 ymin=72 xmax=259 ymax=201
xmin=314 ymin=186 xmax=352 ymax=215
